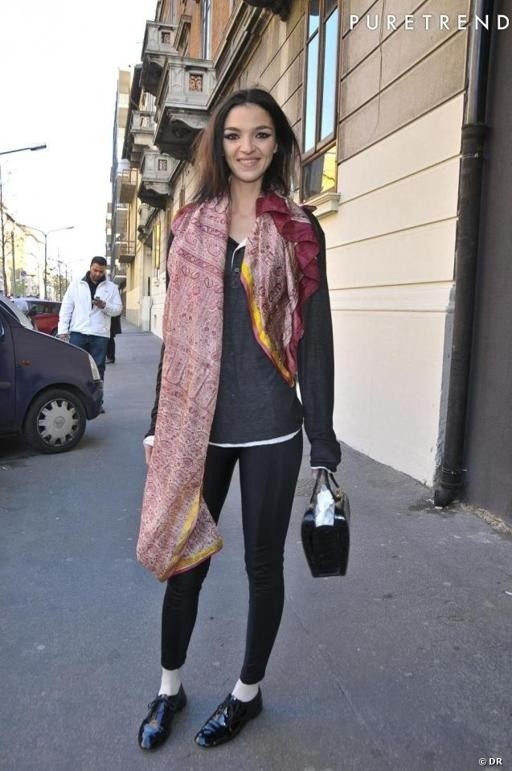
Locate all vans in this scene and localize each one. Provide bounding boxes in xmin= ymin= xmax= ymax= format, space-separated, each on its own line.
xmin=0 ymin=294 xmax=105 ymax=453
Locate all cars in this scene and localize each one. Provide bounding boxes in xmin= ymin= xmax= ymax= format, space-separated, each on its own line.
xmin=11 ymin=299 xmax=61 ymax=336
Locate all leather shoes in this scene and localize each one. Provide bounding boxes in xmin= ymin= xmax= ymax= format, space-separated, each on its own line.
xmin=195 ymin=687 xmax=262 ymax=749
xmin=139 ymin=683 xmax=187 ymax=750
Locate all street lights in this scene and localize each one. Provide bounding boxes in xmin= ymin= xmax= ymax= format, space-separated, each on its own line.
xmin=0 ymin=145 xmax=48 ymax=156
xmin=44 ymin=227 xmax=74 ymax=298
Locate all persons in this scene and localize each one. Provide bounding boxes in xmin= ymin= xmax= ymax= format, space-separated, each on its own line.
xmin=136 ymin=87 xmax=342 ymax=754
xmin=57 ymin=256 xmax=124 ymax=413
xmin=106 ymin=312 xmax=121 ymax=365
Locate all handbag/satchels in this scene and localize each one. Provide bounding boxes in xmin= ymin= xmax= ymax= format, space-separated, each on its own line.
xmin=300 ymin=486 xmax=351 ymax=577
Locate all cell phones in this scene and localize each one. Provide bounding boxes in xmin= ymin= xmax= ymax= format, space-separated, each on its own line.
xmin=96 ymin=296 xmax=100 ymax=299
